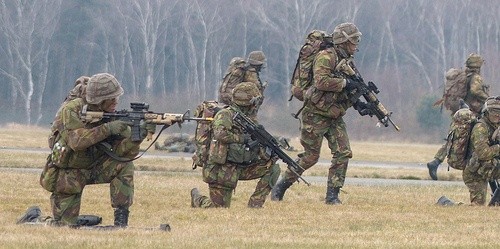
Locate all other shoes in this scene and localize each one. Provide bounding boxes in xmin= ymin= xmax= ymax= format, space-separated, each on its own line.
xmin=434 ymin=196 xmax=452 ymax=206
xmin=486 ymin=186 xmax=500 ymax=206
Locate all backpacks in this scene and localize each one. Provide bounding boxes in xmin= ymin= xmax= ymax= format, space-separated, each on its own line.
xmin=446 ymin=108 xmax=488 ymax=170
xmin=442 ymin=68 xmax=478 ymax=111
xmin=217 ymin=56 xmax=257 ymax=106
xmin=289 ymin=30 xmax=339 ymax=102
xmin=192 ymin=99 xmax=238 ymax=169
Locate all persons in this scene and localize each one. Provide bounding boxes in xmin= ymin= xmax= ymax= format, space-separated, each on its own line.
xmin=427 ymin=53 xmax=500 ymax=206
xmin=17 ymin=74 xmax=170 ymax=231
xmin=271 ymin=23 xmax=374 ymax=205
xmin=155 ymin=135 xmax=196 ymax=152
xmin=191 ymin=81 xmax=281 ymax=208
xmin=235 ymin=51 xmax=267 ymax=117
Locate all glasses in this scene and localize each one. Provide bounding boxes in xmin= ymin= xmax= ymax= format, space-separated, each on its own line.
xmin=486 ymin=108 xmax=500 ymax=115
xmin=347 ymin=36 xmax=361 ymax=45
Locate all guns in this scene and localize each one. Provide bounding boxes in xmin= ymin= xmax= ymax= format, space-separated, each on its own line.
xmin=334 ymin=54 xmax=401 ymax=133
xmin=79 ymin=102 xmax=214 ymax=144
xmin=232 ymin=111 xmax=311 ymax=186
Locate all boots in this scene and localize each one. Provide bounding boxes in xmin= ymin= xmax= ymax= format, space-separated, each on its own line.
xmin=113 ymin=206 xmax=130 ymax=227
xmin=325 ymin=186 xmax=343 ymax=205
xmin=426 ymin=159 xmax=441 ymax=181
xmin=16 ymin=206 xmax=42 ymax=225
xmin=190 ymin=187 xmax=210 ymax=208
xmin=271 ymin=177 xmax=292 ymax=202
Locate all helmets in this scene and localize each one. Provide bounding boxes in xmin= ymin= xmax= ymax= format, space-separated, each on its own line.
xmin=480 ymin=96 xmax=500 ymax=112
xmin=231 ymin=82 xmax=263 ymax=106
xmin=465 ymin=53 xmax=486 ymax=67
xmin=247 ymin=51 xmax=266 ymax=65
xmin=85 ymin=73 xmax=125 ymax=104
xmin=333 ymin=23 xmax=362 ymax=44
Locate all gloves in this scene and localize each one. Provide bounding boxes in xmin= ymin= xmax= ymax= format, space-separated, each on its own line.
xmin=346 ymin=79 xmax=358 ymax=89
xmin=139 ymin=120 xmax=157 ymax=134
xmin=109 ymin=119 xmax=134 ymax=136
xmin=269 ymin=151 xmax=279 ymax=165
xmin=239 ymin=134 xmax=250 ymax=144
xmin=357 ymin=102 xmax=373 ymax=118
